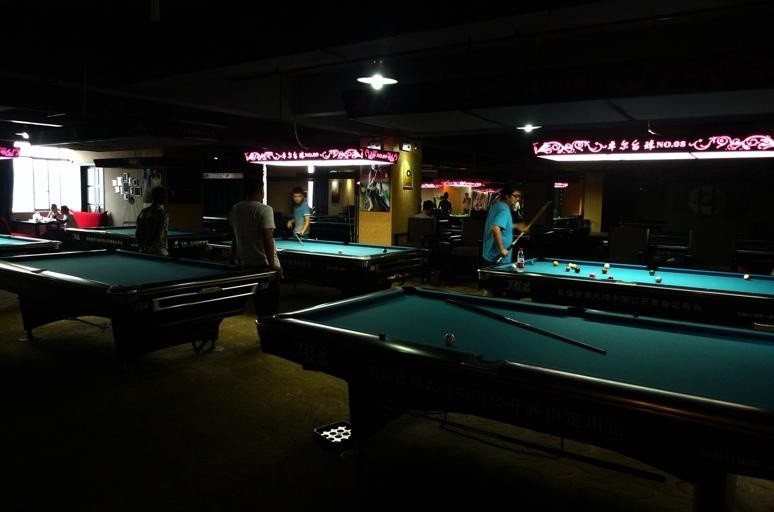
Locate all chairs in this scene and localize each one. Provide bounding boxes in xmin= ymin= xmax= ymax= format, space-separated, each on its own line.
xmin=722 ymin=234 xmax=774 ymax=275
xmin=393 ymin=217 xmax=438 ymax=284
xmin=600 ymin=224 xmax=653 ymax=264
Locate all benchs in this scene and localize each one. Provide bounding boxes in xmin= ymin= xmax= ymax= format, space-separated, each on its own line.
xmin=0 ymin=216 xmax=32 ymax=239
xmin=71 ymin=212 xmax=107 ymax=228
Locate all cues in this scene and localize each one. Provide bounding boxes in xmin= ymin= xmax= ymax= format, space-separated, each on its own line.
xmin=292 ymin=229 xmax=307 ymax=249
xmin=496 ymin=200 xmax=554 ymax=264
xmin=445 ymin=298 xmax=607 ymax=354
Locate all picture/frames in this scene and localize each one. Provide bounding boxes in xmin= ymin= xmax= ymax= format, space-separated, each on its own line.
xmin=111 ymin=172 xmax=142 ymax=201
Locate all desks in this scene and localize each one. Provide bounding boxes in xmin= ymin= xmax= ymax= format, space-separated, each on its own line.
xmin=205 ymin=234 xmax=431 ymax=300
xmin=1 ymin=248 xmax=278 ymax=384
xmin=477 ymin=254 xmax=774 ymax=332
xmin=276 ymin=210 xmax=359 ymax=236
xmin=62 ymin=224 xmax=214 ymax=259
xmin=255 ymin=284 xmax=773 ymax=512
xmin=544 ymin=216 xmax=584 ymax=237
xmin=438 ymin=210 xmax=489 ymax=237
xmin=15 ymin=220 xmax=59 ymax=238
xmin=0 ymin=229 xmax=62 ymax=257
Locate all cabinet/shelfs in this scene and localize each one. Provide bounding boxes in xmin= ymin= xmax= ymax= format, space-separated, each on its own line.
xmin=650 ymin=230 xmax=694 ymax=267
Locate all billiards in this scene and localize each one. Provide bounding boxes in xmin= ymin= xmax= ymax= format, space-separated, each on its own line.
xmin=604 ymin=263 xmax=609 ymax=268
xmin=649 ymin=270 xmax=655 ymax=275
xmin=590 ymin=273 xmax=595 ymax=277
xmin=566 ymin=263 xmax=580 ymax=272
xmin=656 ymin=277 xmax=661 ymax=282
xmin=553 ymin=261 xmax=558 ymax=265
xmin=609 ymin=276 xmax=613 ymax=279
xmin=744 ymin=273 xmax=750 ymax=280
xmin=602 ymin=269 xmax=607 ymax=273
xmin=443 ymin=332 xmax=454 ymax=346
xmin=337 ymin=250 xmax=343 ymax=254
xmin=530 ymin=260 xmax=534 ymax=265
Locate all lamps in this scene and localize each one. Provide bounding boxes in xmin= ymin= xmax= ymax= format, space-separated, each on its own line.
xmin=0 ymin=146 xmax=20 ymax=162
xmin=354 ymin=55 xmax=400 ymax=93
xmin=243 ymin=125 xmax=401 ymax=170
xmin=533 ymin=117 xmax=773 ymax=165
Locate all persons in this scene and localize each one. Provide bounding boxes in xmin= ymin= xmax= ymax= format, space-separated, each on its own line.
xmin=481 ymin=182 xmax=528 ymax=269
xmin=286 ymin=187 xmax=312 ymax=240
xmin=227 ymin=175 xmax=284 ymax=319
xmin=421 ymin=184 xmax=528 ymax=222
xmin=52 ymin=205 xmax=77 ymax=232
xmin=45 ymin=203 xmax=59 ymax=232
xmin=133 ymin=185 xmax=170 ymax=257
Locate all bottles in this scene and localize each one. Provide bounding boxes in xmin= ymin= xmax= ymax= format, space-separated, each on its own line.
xmin=516 ymin=248 xmax=524 ymax=268
